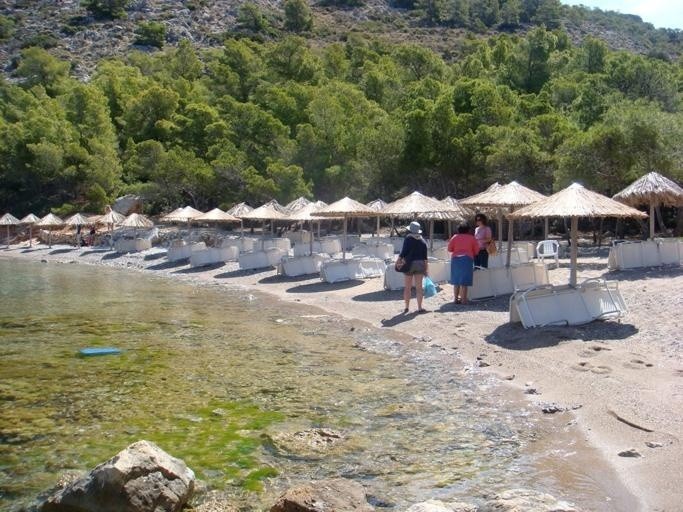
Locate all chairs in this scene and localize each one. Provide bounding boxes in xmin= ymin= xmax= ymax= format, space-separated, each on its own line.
xmin=536 ymin=238 xmax=559 ymax=270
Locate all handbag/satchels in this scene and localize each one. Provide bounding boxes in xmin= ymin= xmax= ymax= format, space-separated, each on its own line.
xmin=487 ymin=240 xmax=497 ymax=257
xmin=396 ymin=257 xmax=411 ymax=275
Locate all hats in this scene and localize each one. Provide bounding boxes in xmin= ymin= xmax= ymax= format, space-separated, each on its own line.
xmin=405 ymin=221 xmax=423 ymax=234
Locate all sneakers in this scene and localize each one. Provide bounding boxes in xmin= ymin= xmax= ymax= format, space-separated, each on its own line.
xmin=454 ymin=299 xmax=468 ymax=305
xmin=404 ymin=308 xmax=426 ymax=314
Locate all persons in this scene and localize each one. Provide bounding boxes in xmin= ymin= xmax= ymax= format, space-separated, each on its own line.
xmin=397 ymin=221 xmax=429 ymax=313
xmin=474 ymin=214 xmax=492 ymax=269
xmin=448 ymin=221 xmax=480 ymax=305
